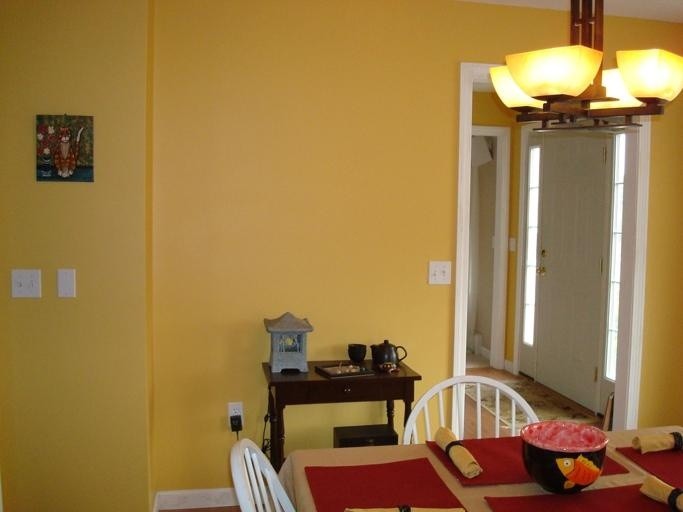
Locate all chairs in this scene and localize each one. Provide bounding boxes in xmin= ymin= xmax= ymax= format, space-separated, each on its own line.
xmin=400 ymin=375 xmax=536 ymax=449
xmin=223 ymin=438 xmax=294 ymax=512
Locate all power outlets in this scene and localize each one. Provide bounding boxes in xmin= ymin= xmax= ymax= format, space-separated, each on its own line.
xmin=227 ymin=403 xmax=245 ymax=432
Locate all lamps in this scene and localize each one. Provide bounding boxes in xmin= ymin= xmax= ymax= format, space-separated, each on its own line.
xmin=483 ymin=2 xmax=682 ymax=130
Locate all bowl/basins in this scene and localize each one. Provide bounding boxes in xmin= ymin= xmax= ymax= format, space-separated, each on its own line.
xmin=520 ymin=421 xmax=607 ymax=495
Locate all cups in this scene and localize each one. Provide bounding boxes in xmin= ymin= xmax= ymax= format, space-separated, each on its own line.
xmin=348 ymin=342 xmax=365 ymax=363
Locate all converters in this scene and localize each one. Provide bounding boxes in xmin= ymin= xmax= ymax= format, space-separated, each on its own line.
xmin=230 ymin=415 xmax=243 ymax=432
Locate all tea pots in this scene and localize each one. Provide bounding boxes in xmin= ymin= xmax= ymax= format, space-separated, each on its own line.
xmin=368 ymin=340 xmax=406 ymax=371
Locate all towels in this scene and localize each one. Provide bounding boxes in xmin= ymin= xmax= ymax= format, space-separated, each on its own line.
xmin=624 ymin=429 xmax=680 ymax=460
xmin=341 ymin=506 xmax=471 ymax=512
xmin=638 ymin=470 xmax=681 ymax=510
xmin=432 ymin=425 xmax=483 ymax=479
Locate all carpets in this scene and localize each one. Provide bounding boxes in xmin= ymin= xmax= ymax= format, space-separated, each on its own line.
xmin=463 ymin=373 xmax=606 ymax=433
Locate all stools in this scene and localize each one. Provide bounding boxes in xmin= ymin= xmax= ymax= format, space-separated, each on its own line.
xmin=329 ymin=423 xmax=399 ymax=449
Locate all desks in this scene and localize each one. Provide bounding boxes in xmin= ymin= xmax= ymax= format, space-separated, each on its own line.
xmin=259 ymin=352 xmax=424 ymax=472
